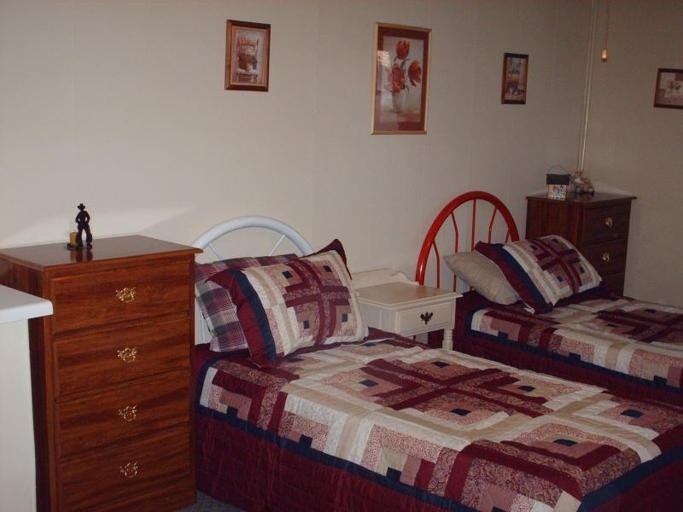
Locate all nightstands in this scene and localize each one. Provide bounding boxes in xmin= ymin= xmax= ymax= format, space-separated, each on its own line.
xmin=350 ymin=267 xmax=463 ymax=353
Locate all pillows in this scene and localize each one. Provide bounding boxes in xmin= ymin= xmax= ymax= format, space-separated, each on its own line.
xmin=192 ymin=252 xmax=301 ymax=357
xmin=202 ymin=237 xmax=369 ymax=370
xmin=437 ymin=250 xmax=520 ymax=306
xmin=473 ymin=233 xmax=608 ymax=316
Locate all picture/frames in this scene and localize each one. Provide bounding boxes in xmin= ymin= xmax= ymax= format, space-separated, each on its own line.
xmin=653 ymin=67 xmax=683 ymax=110
xmin=225 ymin=18 xmax=271 ymax=93
xmin=501 ymin=52 xmax=529 ymax=105
xmin=371 ymin=21 xmax=432 ymax=136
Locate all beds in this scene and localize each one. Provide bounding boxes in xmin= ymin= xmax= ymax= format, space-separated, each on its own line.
xmin=413 ymin=189 xmax=683 ymax=411
xmin=188 ymin=216 xmax=683 ymax=508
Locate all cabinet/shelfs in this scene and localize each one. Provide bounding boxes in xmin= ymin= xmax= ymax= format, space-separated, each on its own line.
xmin=522 ymin=184 xmax=636 ymax=302
xmin=0 ymin=233 xmax=207 ymax=512
xmin=0 ymin=282 xmax=54 ymax=512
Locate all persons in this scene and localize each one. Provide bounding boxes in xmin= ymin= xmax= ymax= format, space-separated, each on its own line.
xmin=75 ymin=203 xmax=93 ymax=249
